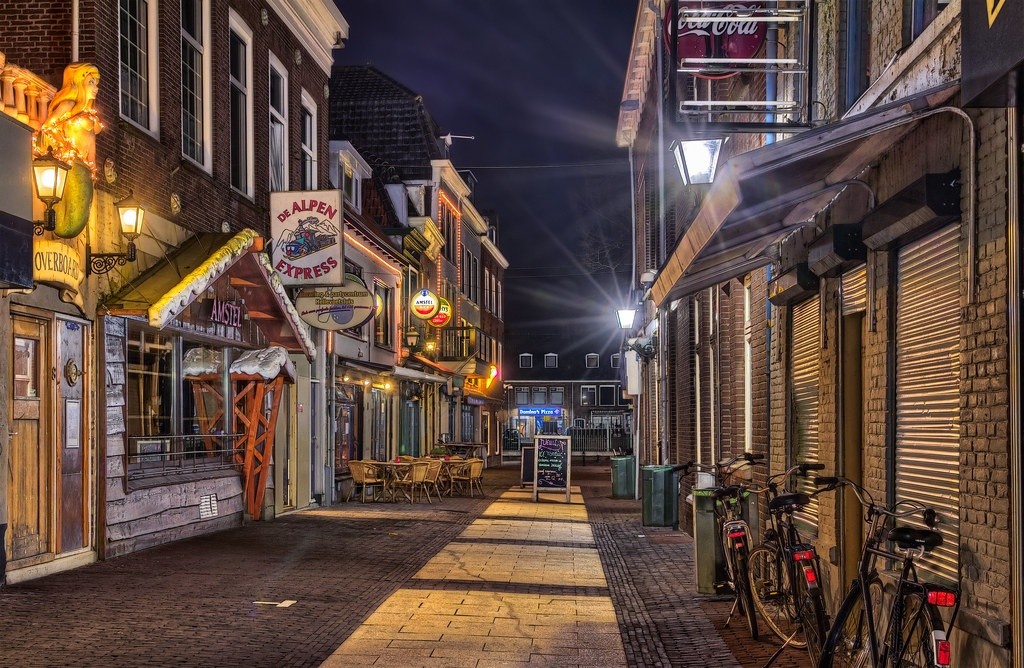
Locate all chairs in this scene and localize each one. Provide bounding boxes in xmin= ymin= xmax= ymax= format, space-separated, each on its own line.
xmin=346 ymin=455 xmax=485 ymax=505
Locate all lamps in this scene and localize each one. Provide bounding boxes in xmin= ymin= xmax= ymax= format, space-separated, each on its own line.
xmin=87 ymin=189 xmax=146 ymax=278
xmin=399 ymin=325 xmax=437 ymax=354
xmin=668 ymin=135 xmax=730 ymax=186
xmin=32 ymin=144 xmax=71 ymax=236
xmin=614 ymin=308 xmax=657 ymax=359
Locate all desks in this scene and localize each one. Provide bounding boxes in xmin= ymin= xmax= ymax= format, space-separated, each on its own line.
xmin=434 ymin=442 xmax=488 ymax=460
xmin=425 ymin=459 xmax=468 ymax=497
xmin=363 ymin=462 xmax=412 ymax=503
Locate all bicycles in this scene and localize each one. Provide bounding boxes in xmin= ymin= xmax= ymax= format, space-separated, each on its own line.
xmin=712 ymin=462 xmax=834 ymax=668
xmin=668 ymin=452 xmax=765 ymax=640
xmin=810 ymin=476 xmax=961 ymax=668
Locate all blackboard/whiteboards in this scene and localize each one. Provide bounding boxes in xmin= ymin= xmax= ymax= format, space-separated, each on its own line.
xmin=520 ymin=447 xmax=535 ymax=485
xmin=533 ymin=435 xmax=572 ymax=492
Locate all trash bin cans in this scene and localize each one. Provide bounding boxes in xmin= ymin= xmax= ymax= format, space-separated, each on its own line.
xmin=693 ymin=487 xmax=747 ymax=601
xmin=641 ymin=464 xmax=681 ymax=530
xmin=608 ymin=456 xmax=637 ymax=500
